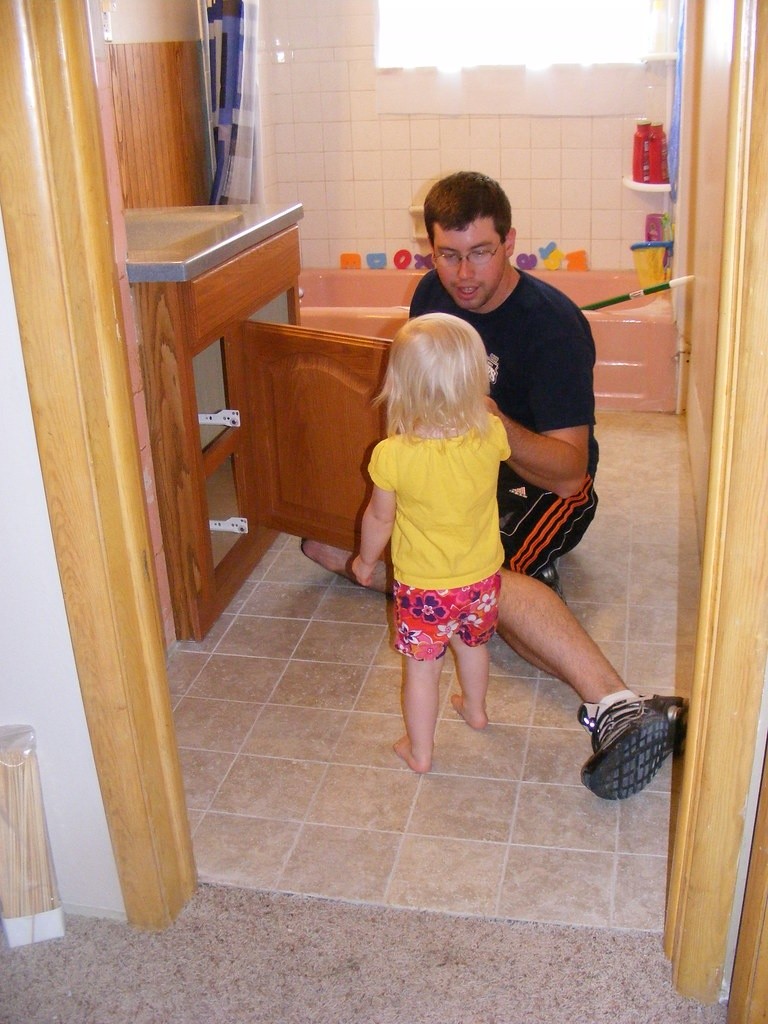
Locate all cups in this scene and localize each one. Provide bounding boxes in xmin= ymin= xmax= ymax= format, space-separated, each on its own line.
xmin=631 ymin=242 xmax=672 ymax=296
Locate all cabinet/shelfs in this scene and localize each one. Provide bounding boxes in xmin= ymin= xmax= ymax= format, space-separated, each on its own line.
xmin=120 ymin=222 xmax=396 ymax=645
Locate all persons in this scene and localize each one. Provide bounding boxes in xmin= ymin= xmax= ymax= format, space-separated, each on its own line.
xmin=302 ymin=171 xmax=689 ymax=799
xmin=353 ymin=312 xmax=513 ymax=773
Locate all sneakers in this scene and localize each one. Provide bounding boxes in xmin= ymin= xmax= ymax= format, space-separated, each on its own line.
xmin=576 ymin=695 xmax=685 ymax=802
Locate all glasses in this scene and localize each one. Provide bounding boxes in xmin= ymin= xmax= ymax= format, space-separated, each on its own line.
xmin=432 ymin=234 xmax=506 ymax=268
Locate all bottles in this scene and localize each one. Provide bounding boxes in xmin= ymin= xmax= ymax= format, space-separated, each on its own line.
xmin=632 ymin=118 xmax=669 ymax=184
xmin=646 ymin=210 xmax=668 ymax=267
